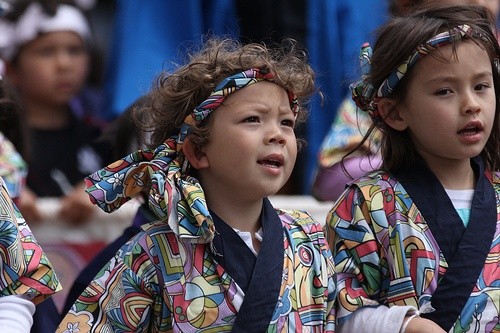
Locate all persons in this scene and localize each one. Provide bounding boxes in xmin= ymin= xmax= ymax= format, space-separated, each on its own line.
xmin=0 ymin=177 xmax=63 ymax=333
xmin=1 ymin=0 xmax=110 ymax=227
xmin=54 ymin=38 xmax=338 ymax=333
xmin=310 ymin=0 xmax=500 ymax=202
xmin=321 ymin=4 xmax=500 ymax=333
